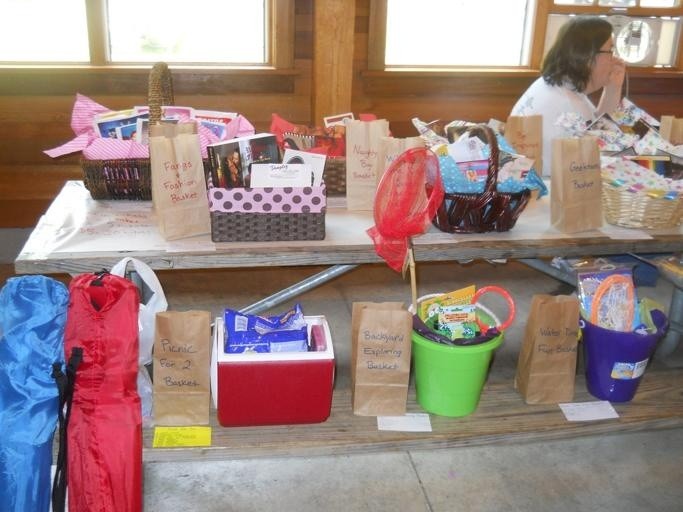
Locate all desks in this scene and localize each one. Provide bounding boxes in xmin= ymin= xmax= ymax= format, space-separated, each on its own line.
xmin=14 ymin=159 xmax=683 ymax=451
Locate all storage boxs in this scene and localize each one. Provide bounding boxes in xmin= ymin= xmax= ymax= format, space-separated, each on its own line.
xmin=217 ymin=314 xmax=335 ymax=430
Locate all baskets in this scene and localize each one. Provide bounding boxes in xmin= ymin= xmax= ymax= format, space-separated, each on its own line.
xmin=602 ymin=189 xmax=683 ymax=229
xmin=80 ymin=63 xmax=214 ymax=200
xmin=426 ymin=126 xmax=530 ymax=233
xmin=322 ymin=157 xmax=346 ymax=193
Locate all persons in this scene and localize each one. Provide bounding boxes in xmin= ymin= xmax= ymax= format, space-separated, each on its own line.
xmin=510 ymin=16 xmax=626 ymax=177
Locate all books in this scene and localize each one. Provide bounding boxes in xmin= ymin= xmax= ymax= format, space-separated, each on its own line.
xmin=93 ymin=105 xmax=355 ymax=188
